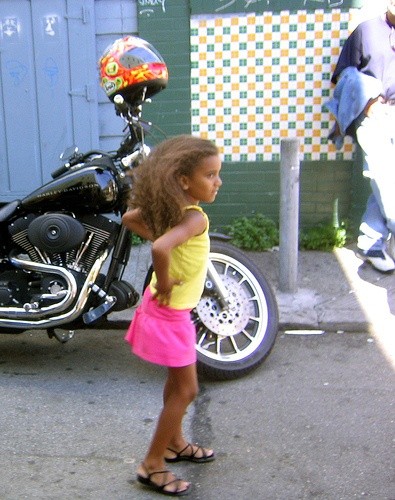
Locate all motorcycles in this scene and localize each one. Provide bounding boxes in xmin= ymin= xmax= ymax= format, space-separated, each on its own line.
xmin=0 ymin=85 xmax=279 ymax=381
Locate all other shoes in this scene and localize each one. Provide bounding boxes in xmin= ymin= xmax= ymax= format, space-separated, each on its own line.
xmin=356 ymin=250 xmax=395 ymax=274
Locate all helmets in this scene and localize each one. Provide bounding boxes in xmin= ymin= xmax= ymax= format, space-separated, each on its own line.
xmin=97 ymin=34 xmax=168 ymax=103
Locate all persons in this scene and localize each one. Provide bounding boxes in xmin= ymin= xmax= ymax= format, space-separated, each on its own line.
xmin=120 ymin=135 xmax=223 ymax=497
xmin=330 ymin=1 xmax=395 ymax=274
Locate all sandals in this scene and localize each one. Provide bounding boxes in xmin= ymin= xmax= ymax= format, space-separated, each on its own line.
xmin=135 ymin=462 xmax=193 ymax=496
xmin=164 ymin=443 xmax=216 ymax=462
